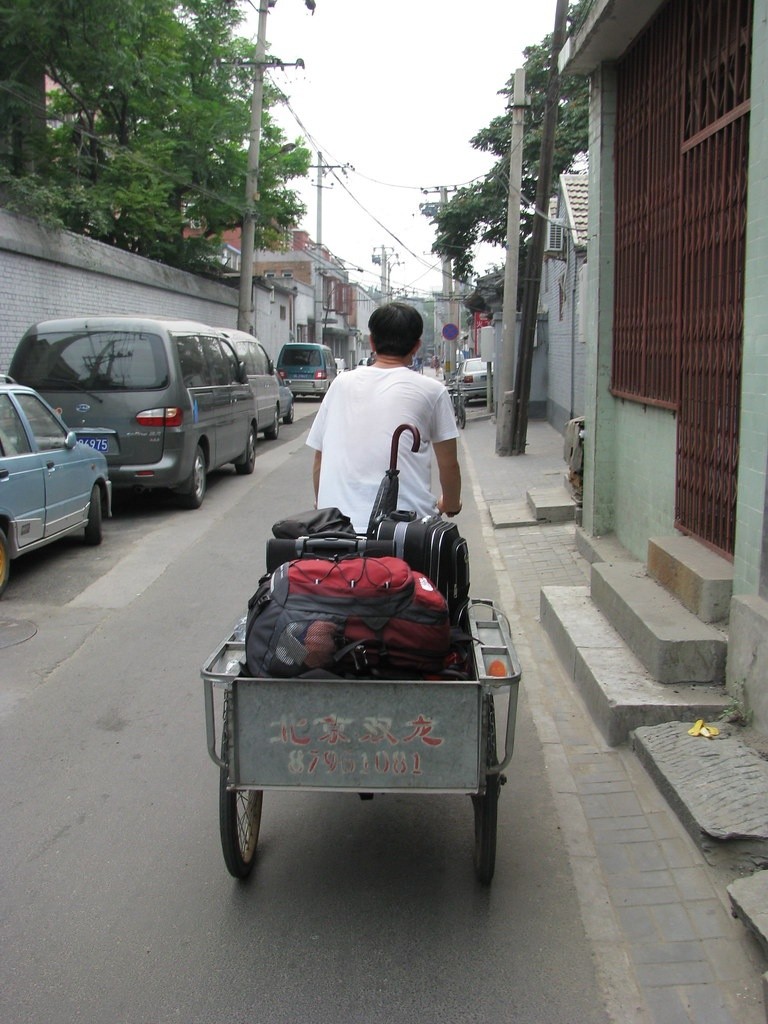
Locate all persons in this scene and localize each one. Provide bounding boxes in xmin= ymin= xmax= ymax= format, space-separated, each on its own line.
xmin=434 ymin=356 xmax=440 ymax=375
xmin=367 ymin=352 xmax=376 ymax=366
xmin=415 ymin=356 xmax=423 ymax=374
xmin=305 ymin=302 xmax=463 ymax=541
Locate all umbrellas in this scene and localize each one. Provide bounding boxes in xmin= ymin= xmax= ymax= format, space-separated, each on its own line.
xmin=366 ymin=423 xmax=421 ymax=538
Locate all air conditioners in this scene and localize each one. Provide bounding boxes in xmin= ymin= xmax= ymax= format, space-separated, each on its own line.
xmin=542 ymin=218 xmax=564 ymax=252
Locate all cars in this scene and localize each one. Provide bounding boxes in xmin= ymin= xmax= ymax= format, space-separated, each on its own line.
xmin=0 ymin=373 xmax=113 ymax=597
xmin=274 ymin=368 xmax=295 ymax=425
xmin=456 ymin=357 xmax=493 ymax=406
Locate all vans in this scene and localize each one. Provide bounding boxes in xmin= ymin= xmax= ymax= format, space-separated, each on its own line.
xmin=4 ymin=314 xmax=259 ymax=511
xmin=276 ymin=342 xmax=338 ymax=401
xmin=210 ymin=326 xmax=282 ymax=440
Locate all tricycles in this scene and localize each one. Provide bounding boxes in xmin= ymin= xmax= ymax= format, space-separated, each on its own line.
xmin=199 ymin=491 xmax=525 ymax=890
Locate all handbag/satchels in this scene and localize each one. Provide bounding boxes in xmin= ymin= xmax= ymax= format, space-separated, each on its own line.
xmin=267 ymin=536 xmax=398 ymax=578
xmin=272 ymin=508 xmax=358 ymax=537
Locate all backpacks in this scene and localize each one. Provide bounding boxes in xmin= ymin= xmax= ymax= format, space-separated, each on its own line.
xmin=245 ymin=556 xmax=451 ymax=678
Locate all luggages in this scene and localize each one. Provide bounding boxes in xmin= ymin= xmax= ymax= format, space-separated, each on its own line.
xmin=367 ymin=511 xmax=467 ymax=624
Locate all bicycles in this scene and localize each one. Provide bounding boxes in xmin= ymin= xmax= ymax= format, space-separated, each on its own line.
xmin=444 ymin=379 xmax=471 ymax=430
